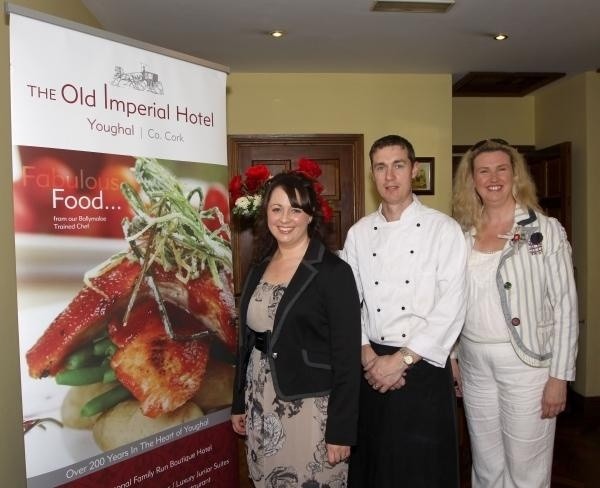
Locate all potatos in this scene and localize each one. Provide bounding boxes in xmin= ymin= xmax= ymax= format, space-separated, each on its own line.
xmin=60 ymin=380 xmax=120 ymax=430
xmin=92 ymin=400 xmax=205 ymax=453
xmin=193 ymin=358 xmax=236 ymax=408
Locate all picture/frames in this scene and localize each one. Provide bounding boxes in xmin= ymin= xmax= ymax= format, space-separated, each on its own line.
xmin=412 ymin=157 xmax=435 ymax=195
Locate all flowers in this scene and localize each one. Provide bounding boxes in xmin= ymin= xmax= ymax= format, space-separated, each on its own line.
xmin=227 ymin=156 xmax=335 ymax=239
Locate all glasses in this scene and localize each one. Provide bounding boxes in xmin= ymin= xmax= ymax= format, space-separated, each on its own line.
xmin=470 ymin=138 xmax=509 ymax=152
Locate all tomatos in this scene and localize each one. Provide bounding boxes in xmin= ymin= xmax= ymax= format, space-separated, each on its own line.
xmin=12 ymin=145 xmax=230 ymax=240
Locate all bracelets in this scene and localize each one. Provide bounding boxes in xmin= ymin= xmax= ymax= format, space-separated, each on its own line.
xmin=398 ymin=348 xmax=415 ymax=369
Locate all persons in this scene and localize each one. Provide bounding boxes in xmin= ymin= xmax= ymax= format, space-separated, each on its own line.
xmin=443 ymin=137 xmax=583 ymax=488
xmin=232 ymin=171 xmax=363 ymax=486
xmin=333 ymin=133 xmax=471 ymax=486
